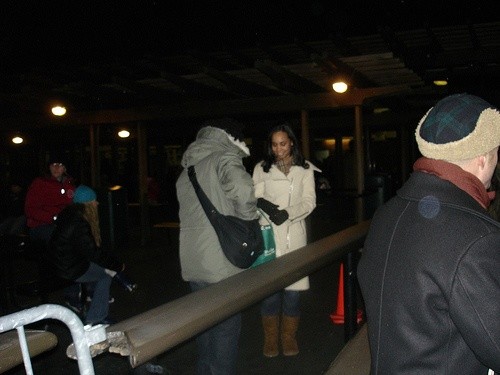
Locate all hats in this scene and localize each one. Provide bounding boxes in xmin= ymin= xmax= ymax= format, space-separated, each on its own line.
xmin=415 ymin=94 xmax=500 ymax=164
xmin=48 ymin=155 xmax=68 ymax=168
xmin=73 ymin=185 xmax=97 ymax=204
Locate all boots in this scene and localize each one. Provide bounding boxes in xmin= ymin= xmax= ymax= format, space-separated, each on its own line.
xmin=261 ymin=315 xmax=280 ymax=358
xmin=279 ymin=312 xmax=301 ymax=356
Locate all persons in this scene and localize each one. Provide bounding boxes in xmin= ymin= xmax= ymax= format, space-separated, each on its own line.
xmin=358 ymin=93 xmax=500 ymax=375
xmin=175 ymin=113 xmax=260 ymax=375
xmin=253 ymin=124 xmax=317 ymax=357
xmin=144 ymin=172 xmax=160 ymax=203
xmin=48 ymin=184 xmax=136 ymax=325
xmin=24 ymin=151 xmax=74 ymax=239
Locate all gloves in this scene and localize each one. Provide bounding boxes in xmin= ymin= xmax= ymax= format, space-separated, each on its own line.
xmin=257 ymin=197 xmax=289 ymax=226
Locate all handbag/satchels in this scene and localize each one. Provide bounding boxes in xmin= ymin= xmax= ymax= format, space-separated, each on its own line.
xmin=213 ymin=215 xmax=264 ymax=270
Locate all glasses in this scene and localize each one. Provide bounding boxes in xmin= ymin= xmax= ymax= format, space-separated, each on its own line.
xmin=53 ymin=162 xmax=63 ymax=167
